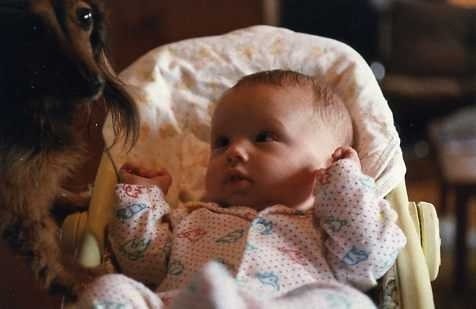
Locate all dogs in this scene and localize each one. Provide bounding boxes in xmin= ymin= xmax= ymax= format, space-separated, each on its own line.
xmin=0 ymin=0 xmax=142 ymax=309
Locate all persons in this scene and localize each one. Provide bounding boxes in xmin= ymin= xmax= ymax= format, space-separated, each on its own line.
xmin=74 ymin=68 xmax=408 ymax=309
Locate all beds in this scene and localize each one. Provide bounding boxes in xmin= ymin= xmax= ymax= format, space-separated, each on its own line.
xmin=60 ymin=25 xmax=442 ymax=309
